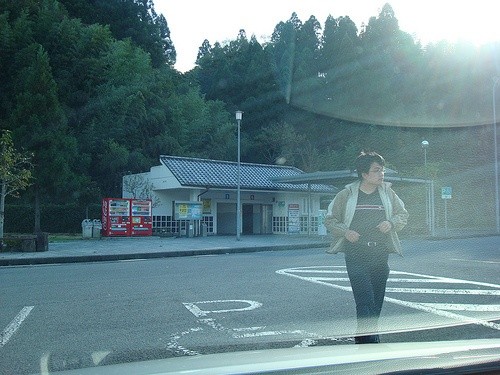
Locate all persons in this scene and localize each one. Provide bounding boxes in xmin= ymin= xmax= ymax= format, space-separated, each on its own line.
xmin=323 ymin=152 xmax=409 ymax=345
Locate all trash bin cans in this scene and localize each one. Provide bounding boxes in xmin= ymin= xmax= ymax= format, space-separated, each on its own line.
xmin=82 ymin=219 xmax=93 ymax=238
xmin=91 ymin=219 xmax=102 ymax=238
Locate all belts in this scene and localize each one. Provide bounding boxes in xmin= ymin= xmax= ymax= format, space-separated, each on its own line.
xmin=348 ymin=240 xmax=388 ymax=247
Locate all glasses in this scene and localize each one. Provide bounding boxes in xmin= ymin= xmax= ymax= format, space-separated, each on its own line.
xmin=368 ymin=167 xmax=386 ymax=173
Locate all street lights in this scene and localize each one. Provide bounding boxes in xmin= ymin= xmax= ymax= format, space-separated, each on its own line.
xmin=234 ymin=108 xmax=245 ymax=241
